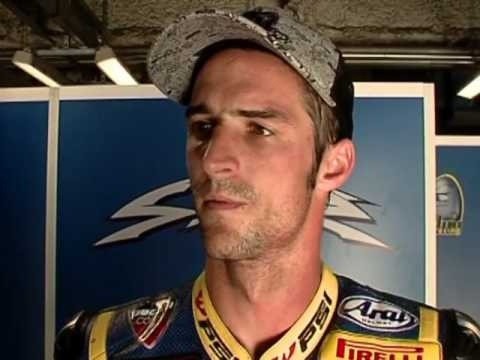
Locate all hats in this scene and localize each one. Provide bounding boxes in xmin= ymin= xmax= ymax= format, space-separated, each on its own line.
xmin=146 ymin=7 xmax=355 ymax=123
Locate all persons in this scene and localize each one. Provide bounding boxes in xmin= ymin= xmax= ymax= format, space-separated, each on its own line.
xmin=54 ymin=5 xmax=479 ymax=360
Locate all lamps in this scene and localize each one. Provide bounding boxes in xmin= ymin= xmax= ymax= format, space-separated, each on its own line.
xmin=95 ymin=45 xmax=139 ymax=85
xmin=12 ymin=48 xmax=63 ymax=87
xmin=457 ymin=67 xmax=480 ymax=99
